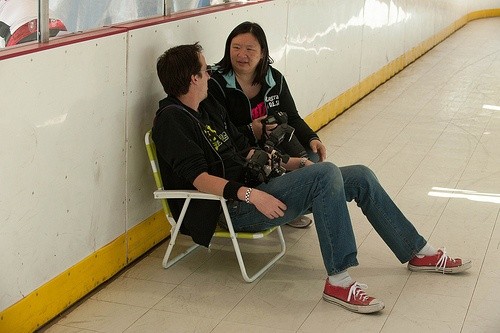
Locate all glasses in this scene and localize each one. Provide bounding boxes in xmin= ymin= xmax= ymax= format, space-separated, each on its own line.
xmin=200 ymin=65 xmax=212 ymax=77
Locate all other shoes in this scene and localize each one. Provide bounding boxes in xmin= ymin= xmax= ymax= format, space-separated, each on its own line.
xmin=288 ymin=215 xmax=312 ymax=228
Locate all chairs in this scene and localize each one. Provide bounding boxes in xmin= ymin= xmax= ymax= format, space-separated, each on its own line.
xmin=145 ymin=130 xmax=286 ymax=282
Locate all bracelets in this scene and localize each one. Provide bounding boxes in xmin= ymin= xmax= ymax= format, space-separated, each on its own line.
xmin=223 ymin=181 xmax=243 ymax=202
xmin=245 ymin=187 xmax=253 ymax=203
xmin=298 ymin=158 xmax=307 ymax=168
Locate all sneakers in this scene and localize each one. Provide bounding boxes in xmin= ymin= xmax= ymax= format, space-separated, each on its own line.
xmin=408 ymin=248 xmax=473 ymax=273
xmin=322 ymin=276 xmax=385 ymax=314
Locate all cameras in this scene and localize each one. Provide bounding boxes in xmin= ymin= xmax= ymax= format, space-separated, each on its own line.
xmin=243 ymin=112 xmax=295 ymax=182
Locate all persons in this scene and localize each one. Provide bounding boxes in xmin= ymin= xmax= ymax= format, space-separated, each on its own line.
xmin=208 ymin=22 xmax=327 ymax=227
xmin=151 ymin=43 xmax=472 ymax=314
